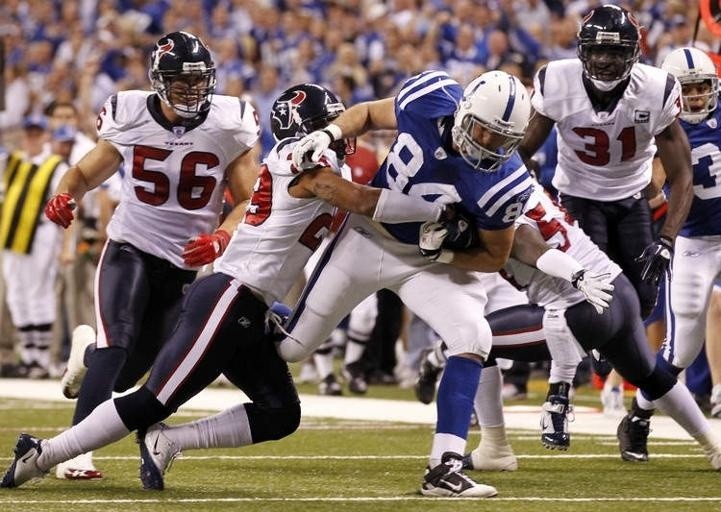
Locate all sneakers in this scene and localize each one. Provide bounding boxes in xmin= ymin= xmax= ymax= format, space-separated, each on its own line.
xmin=136 ymin=422 xmax=182 ymax=491
xmin=64 ymin=468 xmax=102 ymax=480
xmin=2 ymin=434 xmax=50 ymax=488
xmin=420 ymin=452 xmax=498 ymax=496
xmin=61 ymin=326 xmax=97 ymax=399
xmin=599 ymin=382 xmax=628 ymax=419
xmin=618 ymin=415 xmax=650 ymax=463
xmin=463 ymin=450 xmax=517 ymax=470
xmin=414 ymin=349 xmax=443 ymax=404
xmin=540 ymin=396 xmax=576 ymax=450
xmin=320 ymin=361 xmax=367 ymax=395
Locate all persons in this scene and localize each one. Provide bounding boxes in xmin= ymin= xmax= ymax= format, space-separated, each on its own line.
xmin=0 ymin=83 xmax=477 ymax=491
xmin=2 ymin=0 xmax=721 ymax=421
xmin=517 ymin=4 xmax=695 ymax=448
xmin=44 ymin=30 xmax=264 ymax=487
xmin=463 ymin=175 xmax=721 ymax=472
xmin=617 ymin=45 xmax=721 ymax=464
xmin=267 ymin=69 xmax=534 ymax=500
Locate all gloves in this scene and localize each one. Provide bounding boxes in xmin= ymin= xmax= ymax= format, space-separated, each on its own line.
xmin=447 ymin=215 xmax=477 ymax=249
xmin=572 ymin=269 xmax=614 ymax=314
xmin=292 ymin=129 xmax=333 ymax=165
xmin=182 ymin=230 xmax=231 ymax=266
xmin=417 ymin=221 xmax=448 ymax=262
xmin=635 ymin=234 xmax=674 ymax=287
xmin=45 ymin=194 xmax=76 ymax=229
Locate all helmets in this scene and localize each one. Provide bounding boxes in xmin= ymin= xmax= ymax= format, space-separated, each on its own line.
xmin=662 ymin=48 xmax=721 ymax=124
xmin=270 ymin=82 xmax=354 ymax=155
xmin=452 ymin=70 xmax=531 ymax=173
xmin=576 ymin=4 xmax=642 ymax=91
xmin=149 ymin=31 xmax=216 ymax=118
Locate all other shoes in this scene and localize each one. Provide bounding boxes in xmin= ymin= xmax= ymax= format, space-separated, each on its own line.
xmin=501 ymin=384 xmax=526 ymax=403
xmin=5 ymin=360 xmax=50 ymax=379
